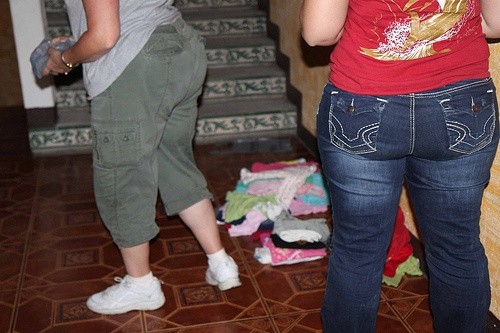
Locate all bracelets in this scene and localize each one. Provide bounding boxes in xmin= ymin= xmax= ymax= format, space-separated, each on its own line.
xmin=61 ymin=51 xmax=75 ymax=68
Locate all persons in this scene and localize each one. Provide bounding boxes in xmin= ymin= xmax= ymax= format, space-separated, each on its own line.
xmin=301 ymin=0 xmax=500 ymax=333
xmin=43 ymin=0 xmax=242 ymax=315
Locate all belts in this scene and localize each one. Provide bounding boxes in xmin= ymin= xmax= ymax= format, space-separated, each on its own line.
xmin=152 ymin=18 xmax=185 ymax=33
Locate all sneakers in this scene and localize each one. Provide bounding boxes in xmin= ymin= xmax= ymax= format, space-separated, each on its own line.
xmin=86 ymin=276 xmax=166 ymax=315
xmin=205 ymin=255 xmax=243 ymax=291
